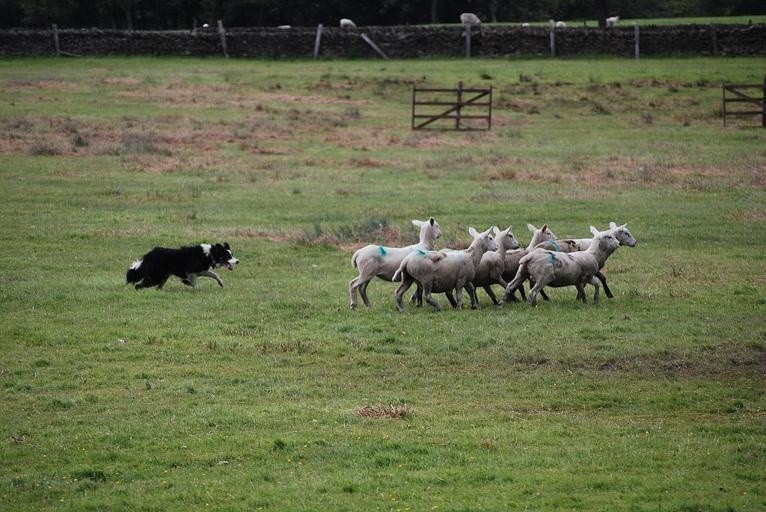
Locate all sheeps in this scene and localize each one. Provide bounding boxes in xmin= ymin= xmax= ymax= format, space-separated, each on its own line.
xmin=349 ymin=216 xmax=637 ymax=314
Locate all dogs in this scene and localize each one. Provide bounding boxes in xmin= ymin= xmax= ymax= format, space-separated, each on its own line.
xmin=126 ymin=242 xmax=240 ymax=291
